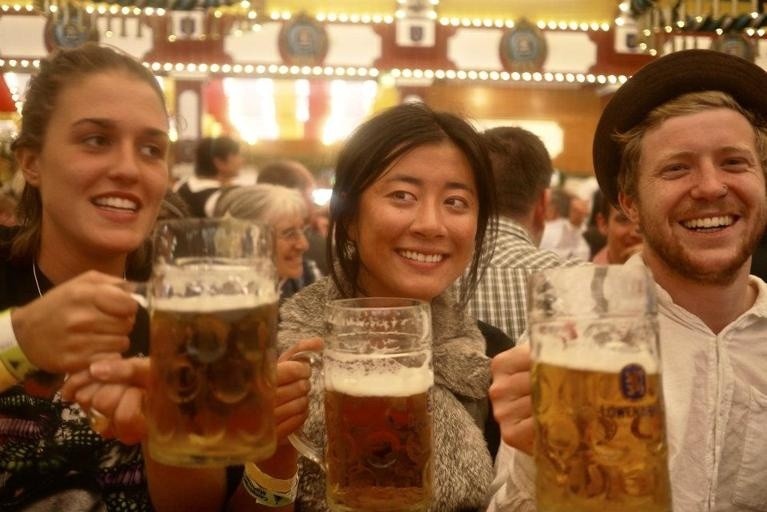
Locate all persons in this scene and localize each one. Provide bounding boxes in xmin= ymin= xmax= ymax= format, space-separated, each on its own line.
xmin=2 ymin=52 xmax=766 ymax=510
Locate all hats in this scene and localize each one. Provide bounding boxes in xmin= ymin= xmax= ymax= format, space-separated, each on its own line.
xmin=591 ymin=49 xmax=741 ymax=215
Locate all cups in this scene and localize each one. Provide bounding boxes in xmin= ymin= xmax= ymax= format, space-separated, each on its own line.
xmin=515 ymin=262 xmax=675 ymax=512
xmin=115 ymin=216 xmax=279 ymax=472
xmin=285 ymin=296 xmax=435 ymax=512
